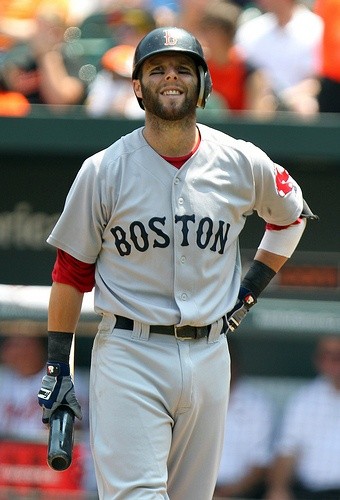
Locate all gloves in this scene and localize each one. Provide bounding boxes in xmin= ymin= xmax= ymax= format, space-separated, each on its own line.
xmin=224 ymin=287 xmax=257 ymax=335
xmin=37 ymin=363 xmax=83 ymax=424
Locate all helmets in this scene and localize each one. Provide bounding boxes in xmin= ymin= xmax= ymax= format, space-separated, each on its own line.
xmin=132 ymin=27 xmax=212 ymax=110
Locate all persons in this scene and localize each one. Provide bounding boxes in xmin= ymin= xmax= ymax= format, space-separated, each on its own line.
xmin=0 ymin=333 xmax=340 ymax=500
xmin=0 ymin=0 xmax=340 ymax=120
xmin=36 ymin=25 xmax=308 ymax=500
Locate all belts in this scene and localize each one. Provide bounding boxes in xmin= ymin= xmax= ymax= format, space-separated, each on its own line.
xmin=114 ymin=314 xmax=226 ymax=340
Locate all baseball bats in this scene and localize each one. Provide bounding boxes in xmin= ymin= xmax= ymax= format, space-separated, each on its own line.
xmin=47 ymin=334 xmax=75 ymax=470
